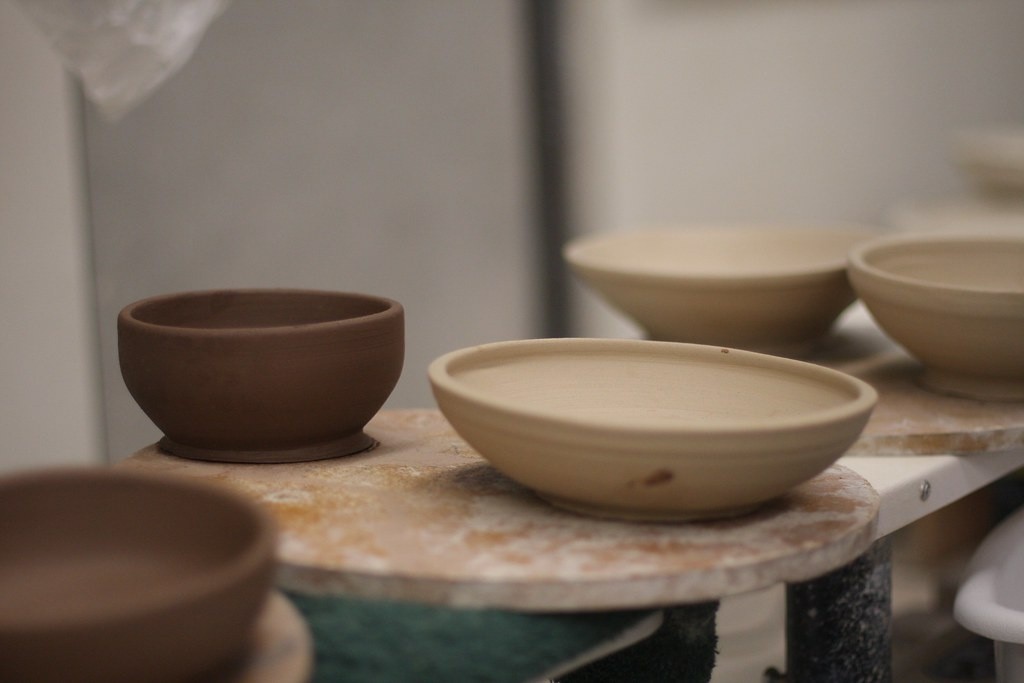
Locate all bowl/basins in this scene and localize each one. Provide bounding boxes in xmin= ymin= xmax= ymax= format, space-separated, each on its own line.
xmin=0 ymin=466 xmax=284 ymax=683
xmin=561 ymin=213 xmax=862 ymax=359
xmin=114 ymin=286 xmax=406 ymax=462
xmin=427 ymin=336 xmax=880 ymax=524
xmin=845 ymin=228 xmax=1024 ymax=401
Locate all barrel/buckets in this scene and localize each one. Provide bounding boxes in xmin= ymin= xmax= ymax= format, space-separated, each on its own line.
xmin=948 ymin=507 xmax=1024 ymax=683
